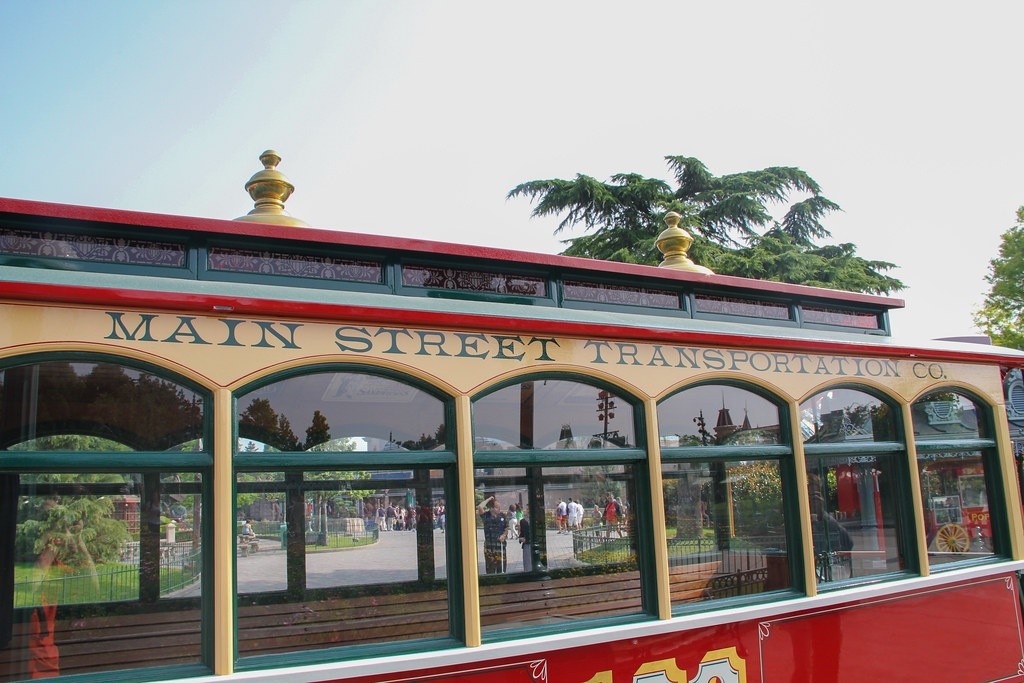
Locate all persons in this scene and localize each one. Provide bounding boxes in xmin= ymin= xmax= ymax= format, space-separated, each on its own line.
xmin=241 ymin=520 xmax=256 ymax=541
xmin=432 ymin=503 xmax=447 ymax=533
xmin=478 ymin=496 xmax=509 ymax=574
xmin=591 ymin=492 xmax=630 ymax=544
xmin=802 ymin=472 xmax=854 ymax=560
xmin=505 ymin=503 xmax=524 ymax=540
xmin=364 ymin=503 xmax=417 ymax=531
xmin=557 ymin=497 xmax=585 ymax=536
xmin=518 ymin=504 xmax=534 ymax=572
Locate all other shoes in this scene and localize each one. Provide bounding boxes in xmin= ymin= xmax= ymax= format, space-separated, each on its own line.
xmin=510 ymin=537 xmax=514 ymax=539
xmin=516 ymin=535 xmax=519 ymax=540
xmin=558 ymin=531 xmax=561 ymax=534
